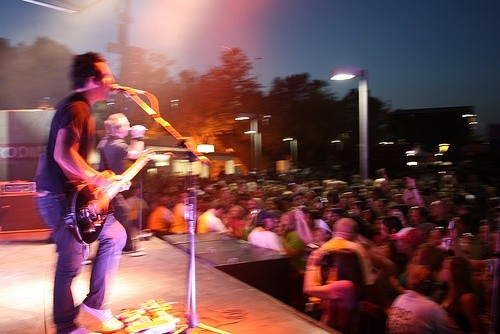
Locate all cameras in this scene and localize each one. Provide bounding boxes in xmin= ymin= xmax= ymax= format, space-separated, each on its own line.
xmin=323 ymin=255 xmax=333 ymax=267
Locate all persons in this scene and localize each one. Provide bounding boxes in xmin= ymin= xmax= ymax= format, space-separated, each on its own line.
xmin=37 ymin=53 xmax=131 ymax=334
xmin=124 ymin=169 xmax=500 ymax=334
xmin=97 ymin=113 xmax=147 ymax=256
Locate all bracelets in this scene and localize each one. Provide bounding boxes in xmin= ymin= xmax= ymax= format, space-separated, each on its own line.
xmin=132 ymin=137 xmax=144 ymax=141
xmin=305 ymin=266 xmax=317 ymax=270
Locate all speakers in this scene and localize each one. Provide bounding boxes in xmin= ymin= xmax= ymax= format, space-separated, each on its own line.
xmin=0 ymin=193 xmax=55 ymax=234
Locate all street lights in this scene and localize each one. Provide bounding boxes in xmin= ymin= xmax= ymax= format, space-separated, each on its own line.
xmin=282 ymin=137 xmax=297 ymax=164
xmin=234 ymin=112 xmax=261 ymax=171
xmin=330 ymin=67 xmax=369 ymax=182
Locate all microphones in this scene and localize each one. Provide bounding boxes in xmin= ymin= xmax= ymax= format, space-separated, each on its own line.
xmin=109 ymin=84 xmax=145 ymax=95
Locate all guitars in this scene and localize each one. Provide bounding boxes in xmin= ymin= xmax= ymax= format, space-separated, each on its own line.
xmin=71 ymin=152 xmax=155 ymax=245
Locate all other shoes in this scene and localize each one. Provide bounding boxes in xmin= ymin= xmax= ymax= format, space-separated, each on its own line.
xmin=78 ymin=304 xmax=125 ymax=332
